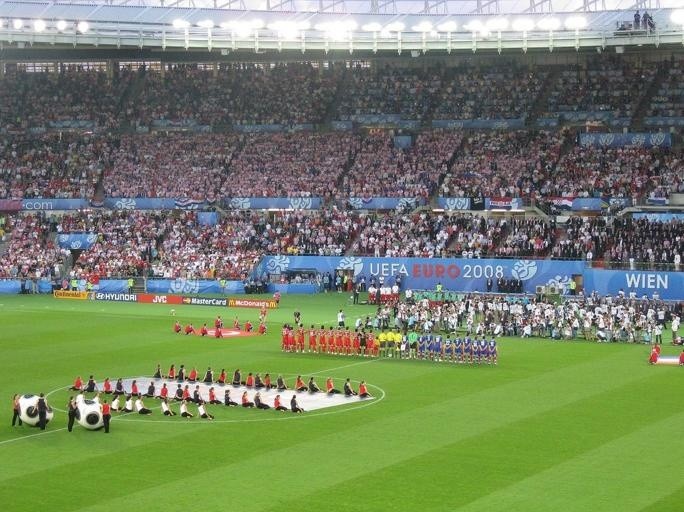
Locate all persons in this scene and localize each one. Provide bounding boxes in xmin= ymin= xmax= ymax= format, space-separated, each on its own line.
xmin=32 ymin=393 xmax=50 ymax=430
xmin=68 ymin=364 xmax=373 ymax=433
xmin=336 ymin=281 xmax=684 ymax=366
xmin=12 ymin=394 xmax=22 ymax=427
xmin=1 ymin=52 xmax=683 ymax=303
xmin=617 ymin=10 xmax=655 ymax=31
xmin=170 ymin=290 xmax=417 ymax=360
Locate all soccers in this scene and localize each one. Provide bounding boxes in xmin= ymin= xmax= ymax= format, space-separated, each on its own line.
xmin=388 ymin=353 xmax=392 ymax=358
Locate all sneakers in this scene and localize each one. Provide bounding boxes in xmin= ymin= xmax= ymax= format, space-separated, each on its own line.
xmin=281 ymin=349 xmax=497 ymax=367
